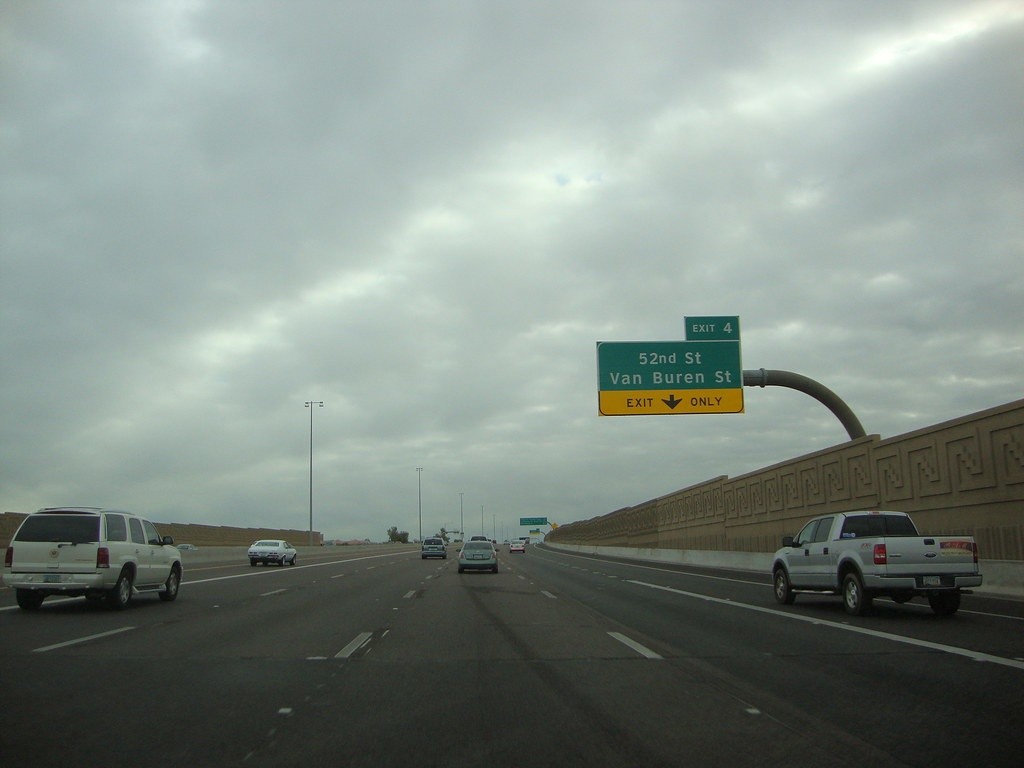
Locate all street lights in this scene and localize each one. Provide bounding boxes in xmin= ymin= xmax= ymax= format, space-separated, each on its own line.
xmin=305 ymin=401 xmax=326 ymax=545
xmin=459 ymin=493 xmax=465 ymax=541
xmin=493 ymin=514 xmax=508 ymax=544
xmin=481 ymin=505 xmax=484 ymax=535
xmin=416 ymin=467 xmax=423 ymax=542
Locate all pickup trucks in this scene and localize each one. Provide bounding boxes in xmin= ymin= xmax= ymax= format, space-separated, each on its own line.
xmin=771 ymin=508 xmax=982 ymax=614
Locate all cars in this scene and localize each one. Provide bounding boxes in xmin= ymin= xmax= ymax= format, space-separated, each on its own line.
xmin=457 ymin=541 xmax=501 ymax=574
xmin=248 ymin=540 xmax=297 ymax=565
xmin=471 ymin=536 xmax=492 ymax=543
xmin=509 ymin=541 xmax=526 ymax=552
xmin=176 ymin=543 xmax=194 ymax=550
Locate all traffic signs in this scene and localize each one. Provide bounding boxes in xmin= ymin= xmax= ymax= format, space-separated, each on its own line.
xmin=520 ymin=517 xmax=548 ymax=525
xmin=530 ymin=530 xmax=541 ymax=536
xmin=596 ymin=342 xmax=746 ymax=417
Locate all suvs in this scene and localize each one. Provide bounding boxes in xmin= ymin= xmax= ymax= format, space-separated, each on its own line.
xmin=421 ymin=538 xmax=448 ymax=559
xmin=2 ymin=505 xmax=183 ymax=610
